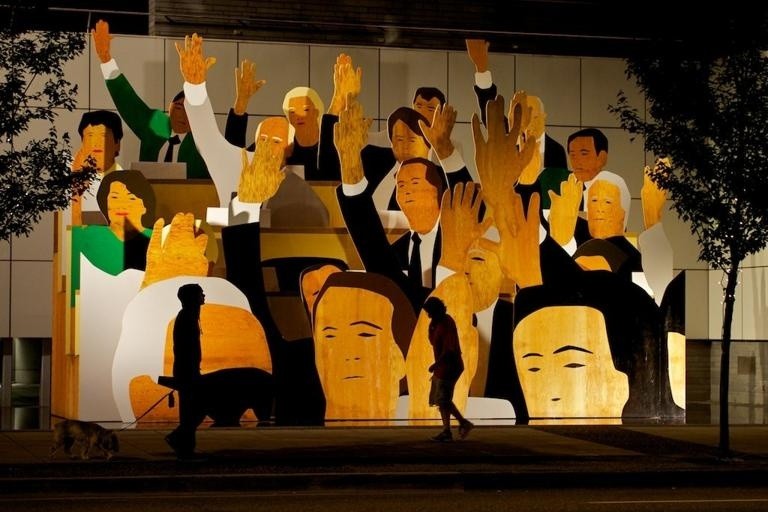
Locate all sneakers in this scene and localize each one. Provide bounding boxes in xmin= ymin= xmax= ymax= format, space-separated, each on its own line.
xmin=430 ymin=419 xmax=474 ymax=443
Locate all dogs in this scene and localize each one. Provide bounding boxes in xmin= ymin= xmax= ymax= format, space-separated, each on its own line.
xmin=49 ymin=420 xmax=120 ymax=461
